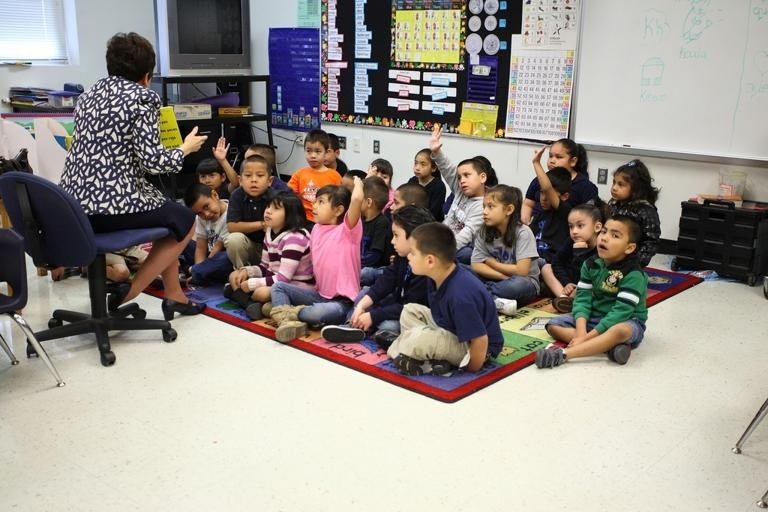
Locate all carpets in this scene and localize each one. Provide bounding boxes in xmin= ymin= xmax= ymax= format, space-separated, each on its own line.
xmin=125 ymin=265 xmax=704 ymax=404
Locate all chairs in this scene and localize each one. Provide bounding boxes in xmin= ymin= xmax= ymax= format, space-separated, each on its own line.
xmin=730 ymin=399 xmax=768 ymax=507
xmin=2 ymin=171 xmax=178 ymax=367
xmin=2 ymin=228 xmax=66 ymax=388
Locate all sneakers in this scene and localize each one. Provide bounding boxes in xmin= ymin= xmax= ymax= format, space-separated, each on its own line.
xmin=392 ymin=353 xmax=452 ymax=377
xmin=149 ymin=273 xmax=186 ymax=290
xmin=607 ymin=344 xmax=631 ymax=365
xmin=536 ymin=343 xmax=567 ymax=369
xmin=552 ymin=296 xmax=573 ymax=314
xmin=494 ymin=297 xmax=517 ymax=316
xmin=320 ymin=324 xmax=364 ymax=343
xmin=373 ymin=329 xmax=399 ymax=352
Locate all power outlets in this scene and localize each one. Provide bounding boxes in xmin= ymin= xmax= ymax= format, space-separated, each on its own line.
xmin=295 ymin=132 xmax=307 ymax=146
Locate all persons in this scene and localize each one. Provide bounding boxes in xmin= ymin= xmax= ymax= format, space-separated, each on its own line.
xmin=529 ymin=145 xmax=573 ymax=297
xmin=520 ymin=137 xmax=599 ymax=226
xmin=457 ymin=182 xmax=539 ymax=319
xmin=540 ymin=203 xmax=604 ymax=314
xmin=385 ymin=221 xmax=504 ymax=379
xmin=173 ymin=121 xmax=502 ymax=350
xmin=607 ymin=158 xmax=662 ymax=270
xmin=56 ymin=31 xmax=208 ymax=321
xmin=47 ymin=225 xmax=157 ymax=296
xmin=536 ymin=214 xmax=649 ymax=370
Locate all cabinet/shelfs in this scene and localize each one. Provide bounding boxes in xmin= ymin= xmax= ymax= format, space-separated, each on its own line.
xmin=151 ymin=74 xmax=278 ymax=205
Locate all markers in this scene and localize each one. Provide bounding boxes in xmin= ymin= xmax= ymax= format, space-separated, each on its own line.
xmin=622 ymin=145 xmax=631 ymax=147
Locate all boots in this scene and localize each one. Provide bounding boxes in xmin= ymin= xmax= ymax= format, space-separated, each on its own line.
xmin=269 ymin=305 xmax=308 ymax=343
xmin=262 ymin=302 xmax=304 ymax=322
xmin=230 ymin=288 xmax=262 ymax=321
xmin=222 ymin=283 xmax=255 ymax=303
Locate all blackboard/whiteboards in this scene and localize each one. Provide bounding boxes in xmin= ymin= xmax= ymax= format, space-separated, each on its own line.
xmin=570 ymin=0 xmax=768 ymax=168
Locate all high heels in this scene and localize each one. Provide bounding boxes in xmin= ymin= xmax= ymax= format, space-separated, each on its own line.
xmin=106 ymin=281 xmax=132 ymax=312
xmin=161 ymin=297 xmax=206 ymax=320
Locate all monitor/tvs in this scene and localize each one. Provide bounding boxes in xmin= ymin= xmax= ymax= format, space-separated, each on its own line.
xmin=153 ymin=0 xmax=252 ymax=77
